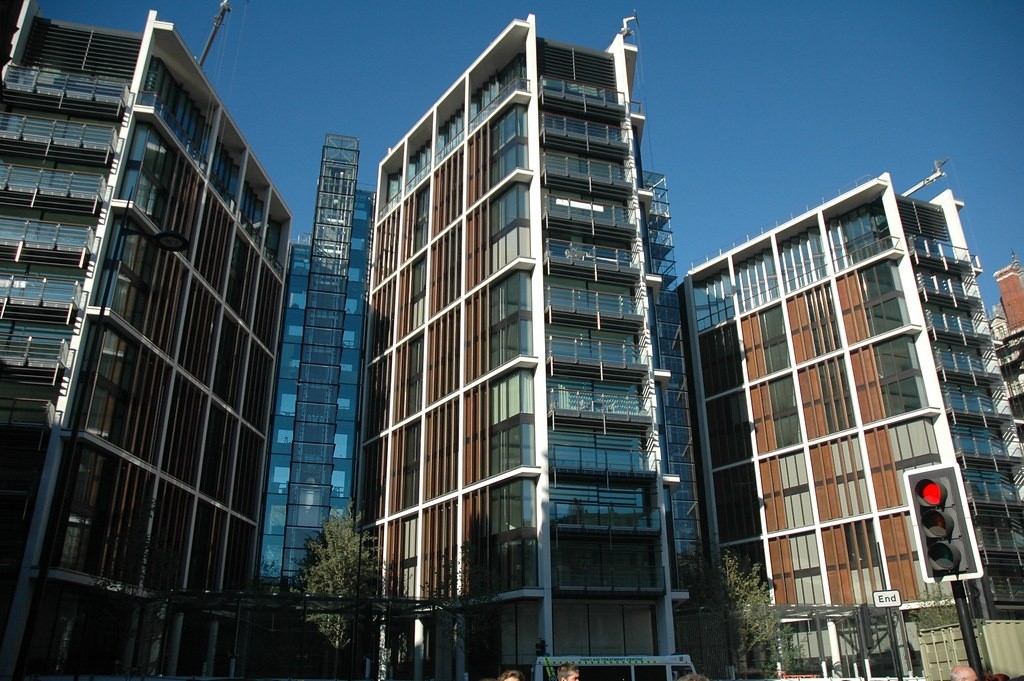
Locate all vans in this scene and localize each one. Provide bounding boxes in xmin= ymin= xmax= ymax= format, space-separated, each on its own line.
xmin=534 ymin=654 xmax=697 ymax=681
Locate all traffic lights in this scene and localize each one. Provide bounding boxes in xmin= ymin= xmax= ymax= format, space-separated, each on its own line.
xmin=904 ymin=462 xmax=984 ymax=582
xmin=534 ymin=637 xmax=546 ymax=656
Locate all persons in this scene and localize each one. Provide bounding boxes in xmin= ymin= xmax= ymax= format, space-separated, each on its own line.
xmin=500 ymin=670 xmax=525 ymax=681
xmin=951 ymin=665 xmax=1011 ymax=681
xmin=558 ymin=663 xmax=579 ymax=681
